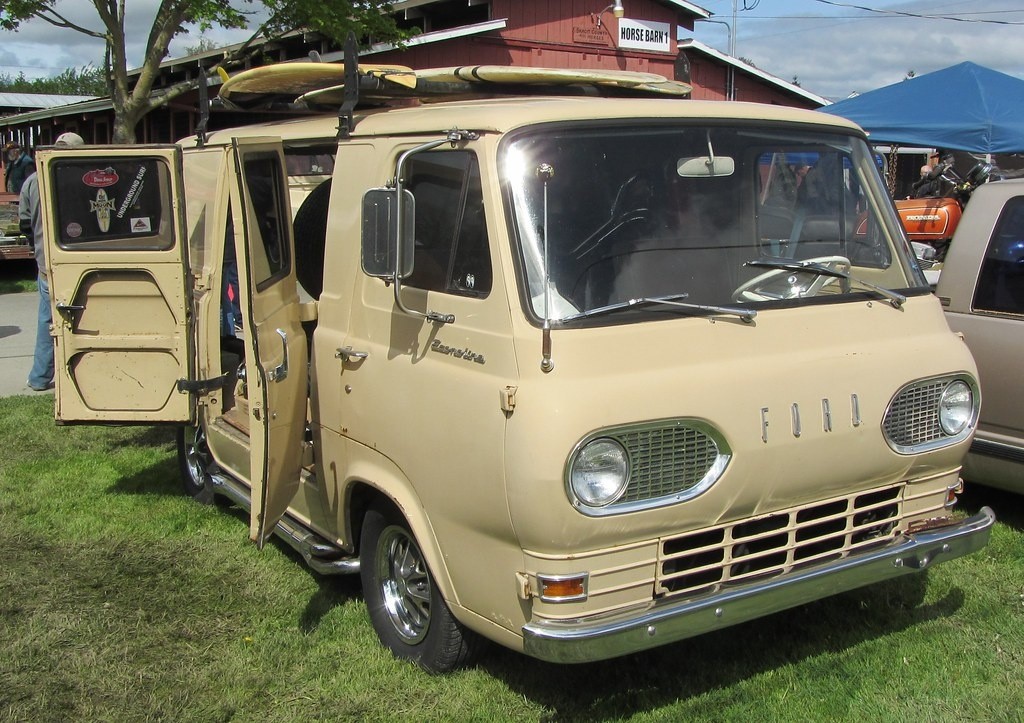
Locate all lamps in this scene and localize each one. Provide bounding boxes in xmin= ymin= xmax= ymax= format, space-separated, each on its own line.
xmin=598 ymin=0 xmax=624 ymax=29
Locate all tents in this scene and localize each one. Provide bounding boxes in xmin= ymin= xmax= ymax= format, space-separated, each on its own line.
xmin=757 ymin=148 xmax=886 ymax=210
xmin=806 ymin=60 xmax=1024 ymax=266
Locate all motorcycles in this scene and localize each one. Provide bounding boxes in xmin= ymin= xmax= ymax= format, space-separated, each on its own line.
xmin=854 ymin=161 xmax=991 ymax=242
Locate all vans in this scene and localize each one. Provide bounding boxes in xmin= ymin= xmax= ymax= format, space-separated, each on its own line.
xmin=32 ymin=30 xmax=995 ymax=676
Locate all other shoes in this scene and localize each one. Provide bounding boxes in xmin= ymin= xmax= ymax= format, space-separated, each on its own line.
xmin=27 ymin=380 xmax=46 ymax=390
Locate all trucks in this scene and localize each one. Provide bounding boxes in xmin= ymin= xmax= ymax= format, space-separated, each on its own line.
xmin=931 ymin=178 xmax=1024 ymax=494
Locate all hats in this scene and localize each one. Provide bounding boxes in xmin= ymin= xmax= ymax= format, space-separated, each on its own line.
xmin=1 ymin=141 xmax=20 ymax=151
xmin=57 ymin=132 xmax=84 ymax=146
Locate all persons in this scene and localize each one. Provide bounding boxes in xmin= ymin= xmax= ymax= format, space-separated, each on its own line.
xmin=16 ymin=132 xmax=87 ymax=391
xmin=906 ymin=159 xmax=948 ymax=201
xmin=2 ymin=140 xmax=36 ymax=193
xmin=792 ymin=163 xmax=827 ymax=215
